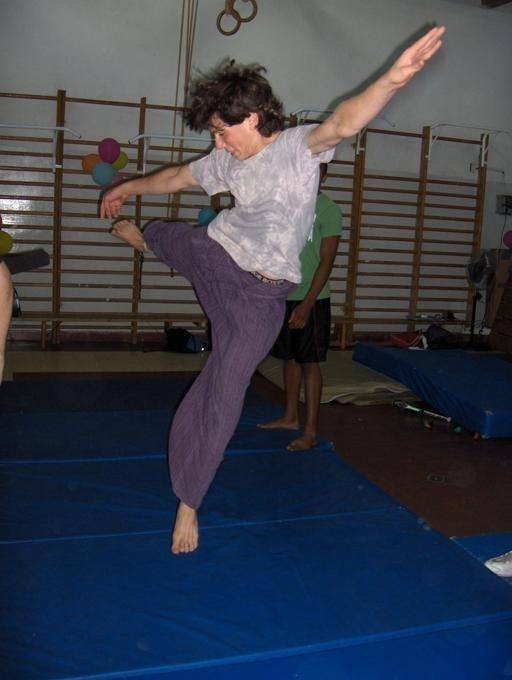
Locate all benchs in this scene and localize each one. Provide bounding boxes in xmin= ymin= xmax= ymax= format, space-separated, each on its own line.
xmin=11 ymin=312 xmax=356 ymax=349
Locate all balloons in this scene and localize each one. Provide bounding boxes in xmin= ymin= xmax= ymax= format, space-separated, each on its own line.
xmin=82 ymin=137 xmax=128 ymax=191
xmin=502 ymin=230 xmax=512 ymax=249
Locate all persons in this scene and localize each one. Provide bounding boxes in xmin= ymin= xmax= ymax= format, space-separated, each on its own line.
xmin=254 ymin=161 xmax=344 ymax=454
xmin=0 ymin=255 xmax=17 ymax=380
xmin=95 ymin=15 xmax=448 ymax=557
